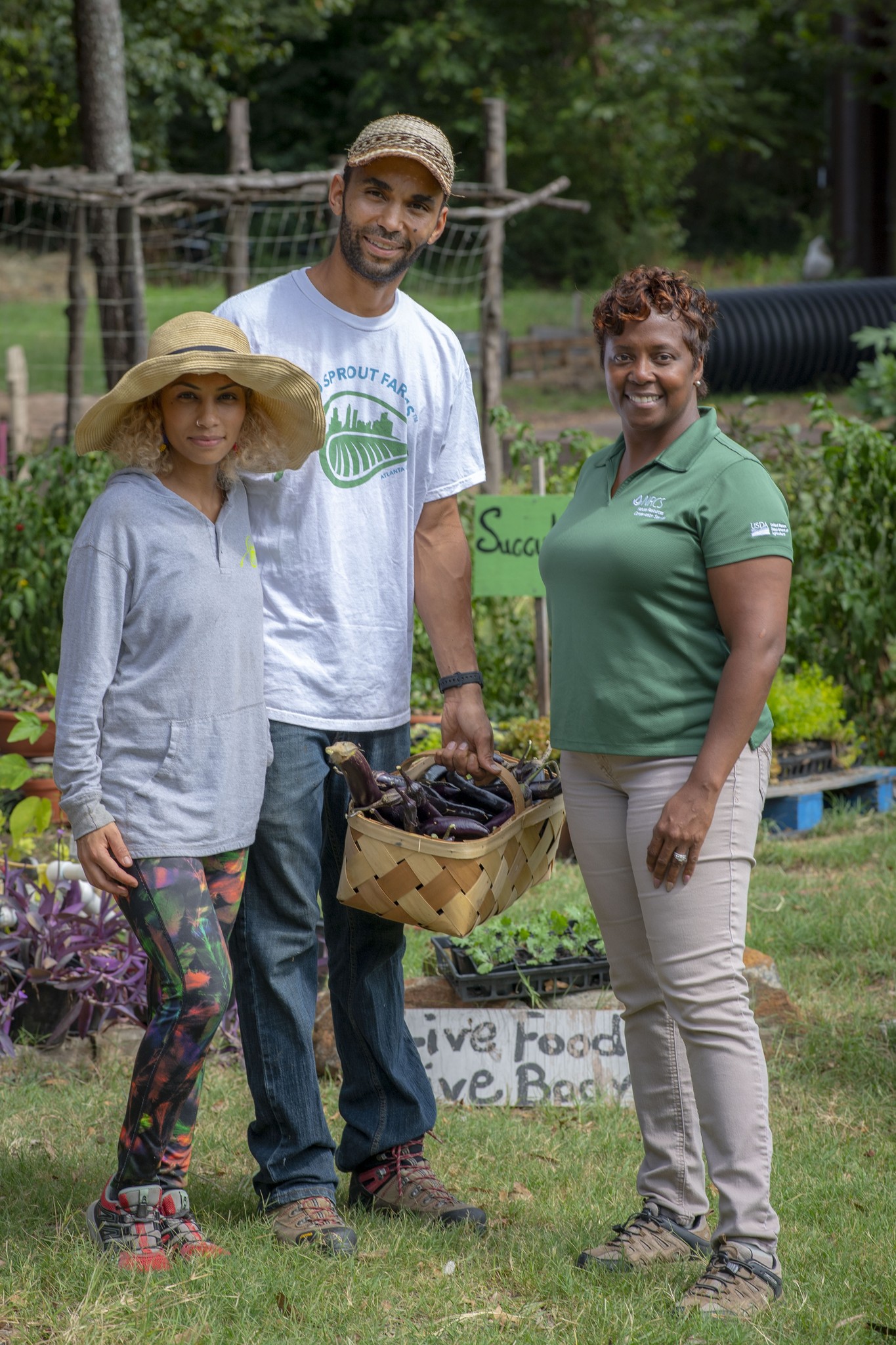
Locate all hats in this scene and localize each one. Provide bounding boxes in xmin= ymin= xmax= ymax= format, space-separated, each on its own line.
xmin=76 ymin=312 xmax=326 ymax=474
xmin=348 ymin=116 xmax=455 ymax=196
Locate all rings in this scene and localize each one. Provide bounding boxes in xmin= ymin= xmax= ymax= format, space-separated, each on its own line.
xmin=672 ymin=851 xmax=686 ymax=862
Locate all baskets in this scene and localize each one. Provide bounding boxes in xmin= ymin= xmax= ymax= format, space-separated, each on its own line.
xmin=334 ymin=750 xmax=564 ymax=937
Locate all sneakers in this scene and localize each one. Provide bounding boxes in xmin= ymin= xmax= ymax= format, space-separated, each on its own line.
xmin=264 ymin=1195 xmax=360 ymax=1263
xmin=152 ymin=1188 xmax=232 ymax=1269
xmin=576 ymin=1201 xmax=708 ymax=1277
xmin=345 ymin=1131 xmax=485 ymax=1232
xmin=677 ymin=1243 xmax=783 ymax=1321
xmin=84 ymin=1174 xmax=172 ymax=1278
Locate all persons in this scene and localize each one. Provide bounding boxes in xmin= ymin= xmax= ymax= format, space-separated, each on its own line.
xmin=538 ymin=264 xmax=793 ymax=1321
xmin=52 ymin=311 xmax=325 ymax=1273
xmin=210 ymin=115 xmax=503 ymax=1257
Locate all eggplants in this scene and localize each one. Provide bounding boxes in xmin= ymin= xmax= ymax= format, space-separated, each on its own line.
xmin=326 ymin=739 xmax=561 ymax=839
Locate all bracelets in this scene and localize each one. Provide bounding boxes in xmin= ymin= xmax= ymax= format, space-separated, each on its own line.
xmin=438 ymin=670 xmax=485 ymax=693
